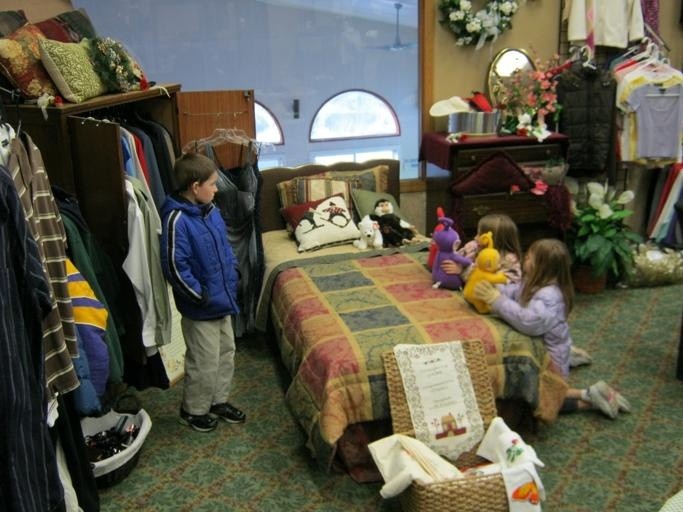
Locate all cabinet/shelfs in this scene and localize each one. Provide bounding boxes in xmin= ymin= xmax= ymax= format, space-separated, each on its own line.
xmin=424 ymin=135 xmax=569 ymax=254
xmin=0 ymin=84 xmax=257 ymax=389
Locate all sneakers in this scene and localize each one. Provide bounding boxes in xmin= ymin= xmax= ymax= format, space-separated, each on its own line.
xmin=179 ymin=402 xmax=219 ymax=431
xmin=615 ymin=392 xmax=631 ymax=413
xmin=210 ymin=403 xmax=246 ymax=424
xmin=587 ymin=379 xmax=617 ymax=419
xmin=568 ymin=345 xmax=592 ymax=368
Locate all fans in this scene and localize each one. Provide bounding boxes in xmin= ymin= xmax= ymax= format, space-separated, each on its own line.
xmin=364 ymin=3 xmax=414 ymax=53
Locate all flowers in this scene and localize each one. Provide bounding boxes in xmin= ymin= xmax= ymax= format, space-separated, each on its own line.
xmin=489 ymin=55 xmax=572 ymax=142
xmin=568 ymin=179 xmax=640 ymax=281
xmin=440 ymin=0 xmax=519 ymax=52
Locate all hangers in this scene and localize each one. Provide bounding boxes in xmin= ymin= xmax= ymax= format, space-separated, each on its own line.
xmin=564 ymin=36 xmax=682 ymax=92
xmin=179 ymin=109 xmax=270 ymax=155
xmin=14 ymin=92 xmax=24 ymax=139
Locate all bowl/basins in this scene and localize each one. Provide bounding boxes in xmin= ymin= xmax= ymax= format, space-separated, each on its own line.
xmin=80 ymin=406 xmax=152 ymax=490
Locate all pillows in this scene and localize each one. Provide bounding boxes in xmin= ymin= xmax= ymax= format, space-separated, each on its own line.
xmin=292 ymin=175 xmax=346 ymax=205
xmin=0 ymin=21 xmax=57 ymax=106
xmin=275 ymin=192 xmax=357 ymax=252
xmin=0 ymin=8 xmax=26 ymax=36
xmin=38 ymin=39 xmax=106 ymax=104
xmin=347 ymin=188 xmax=400 ymax=220
xmin=273 ymin=165 xmax=391 ymax=205
xmin=97 ymin=39 xmax=147 ymax=95
xmin=32 ymin=7 xmax=99 ymax=43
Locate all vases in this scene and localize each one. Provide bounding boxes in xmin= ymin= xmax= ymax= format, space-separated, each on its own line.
xmin=573 ymin=257 xmax=613 ymax=296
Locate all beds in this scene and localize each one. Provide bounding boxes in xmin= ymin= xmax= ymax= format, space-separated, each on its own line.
xmin=251 ymin=158 xmax=571 ymax=485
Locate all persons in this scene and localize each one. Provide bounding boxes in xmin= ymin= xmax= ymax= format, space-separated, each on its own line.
xmin=440 ymin=213 xmax=592 ymax=368
xmin=160 ymin=152 xmax=246 ymax=432
xmin=475 ymin=238 xmax=630 ymax=419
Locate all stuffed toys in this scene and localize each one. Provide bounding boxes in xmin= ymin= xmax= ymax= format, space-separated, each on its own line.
xmin=362 ymin=199 xmax=421 ymax=247
xmin=432 ymin=217 xmax=473 ymax=290
xmin=353 ymin=222 xmax=384 ymax=249
xmin=463 ymin=231 xmax=507 ymax=314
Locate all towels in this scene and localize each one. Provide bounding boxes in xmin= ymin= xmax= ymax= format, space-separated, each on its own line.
xmin=367 ymin=432 xmax=462 ymax=500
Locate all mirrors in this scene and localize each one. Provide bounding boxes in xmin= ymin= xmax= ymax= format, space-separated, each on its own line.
xmin=488 ymin=48 xmax=539 ymax=107
